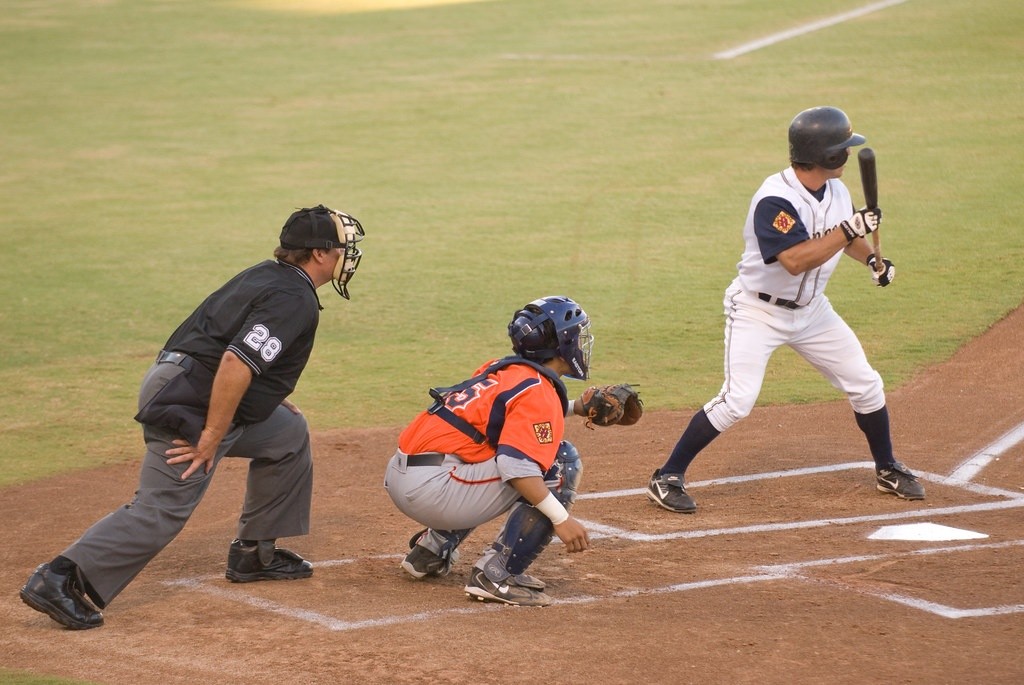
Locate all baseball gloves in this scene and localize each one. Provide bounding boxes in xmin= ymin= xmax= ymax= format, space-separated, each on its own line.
xmin=580 ymin=383 xmax=644 ymax=431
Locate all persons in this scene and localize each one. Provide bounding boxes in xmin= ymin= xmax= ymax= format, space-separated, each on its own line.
xmin=383 ymin=296 xmax=642 ymax=608
xmin=20 ymin=204 xmax=365 ymax=630
xmin=646 ymin=105 xmax=925 ymax=514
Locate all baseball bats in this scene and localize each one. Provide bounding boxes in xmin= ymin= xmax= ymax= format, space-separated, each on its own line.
xmin=858 ymin=147 xmax=883 ymax=272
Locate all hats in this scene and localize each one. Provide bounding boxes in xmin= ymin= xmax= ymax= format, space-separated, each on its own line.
xmin=280 ymin=207 xmax=354 ymax=249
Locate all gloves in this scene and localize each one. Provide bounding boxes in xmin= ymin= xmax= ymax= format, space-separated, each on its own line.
xmin=840 ymin=205 xmax=882 ymax=242
xmin=867 ymin=254 xmax=895 ymax=287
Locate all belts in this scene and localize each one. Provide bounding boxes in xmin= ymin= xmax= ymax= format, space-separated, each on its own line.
xmin=406 ymin=454 xmax=444 ymax=466
xmin=759 ymin=293 xmax=799 ymax=308
xmin=159 ymin=352 xmax=192 ymax=369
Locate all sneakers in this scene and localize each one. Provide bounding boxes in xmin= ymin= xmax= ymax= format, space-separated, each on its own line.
xmin=645 ymin=469 xmax=697 ymax=513
xmin=464 ymin=567 xmax=553 ymax=607
xmin=876 ymin=462 xmax=925 ymax=500
xmin=399 ymin=544 xmax=453 ymax=579
xmin=226 ymin=539 xmax=313 ymax=582
xmin=19 ymin=563 xmax=104 ymax=630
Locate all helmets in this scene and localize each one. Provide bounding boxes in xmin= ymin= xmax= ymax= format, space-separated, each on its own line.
xmin=508 ymin=296 xmax=589 ymax=364
xmin=788 ymin=106 xmax=865 ymax=169
